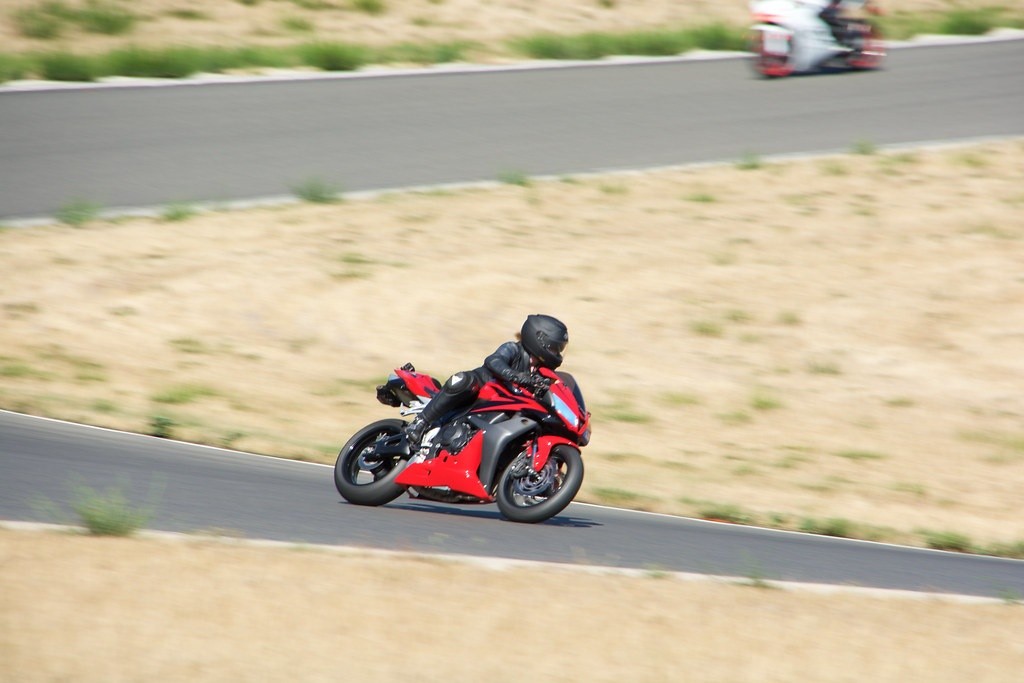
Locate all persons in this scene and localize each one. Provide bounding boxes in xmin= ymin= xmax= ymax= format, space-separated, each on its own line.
xmin=401 ymin=313 xmax=569 ymax=445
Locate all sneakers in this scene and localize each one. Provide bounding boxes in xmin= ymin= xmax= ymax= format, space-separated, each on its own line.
xmin=404 ymin=414 xmax=429 ymax=442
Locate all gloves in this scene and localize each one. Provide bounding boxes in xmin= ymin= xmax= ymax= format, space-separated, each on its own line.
xmin=517 ymin=373 xmax=545 ymax=387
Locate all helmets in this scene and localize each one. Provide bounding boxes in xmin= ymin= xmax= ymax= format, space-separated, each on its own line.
xmin=521 ymin=314 xmax=569 ymax=369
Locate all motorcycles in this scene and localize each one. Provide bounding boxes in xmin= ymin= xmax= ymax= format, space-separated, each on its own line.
xmin=334 ymin=361 xmax=593 ymax=524
xmin=750 ymin=0 xmax=887 ymax=79
